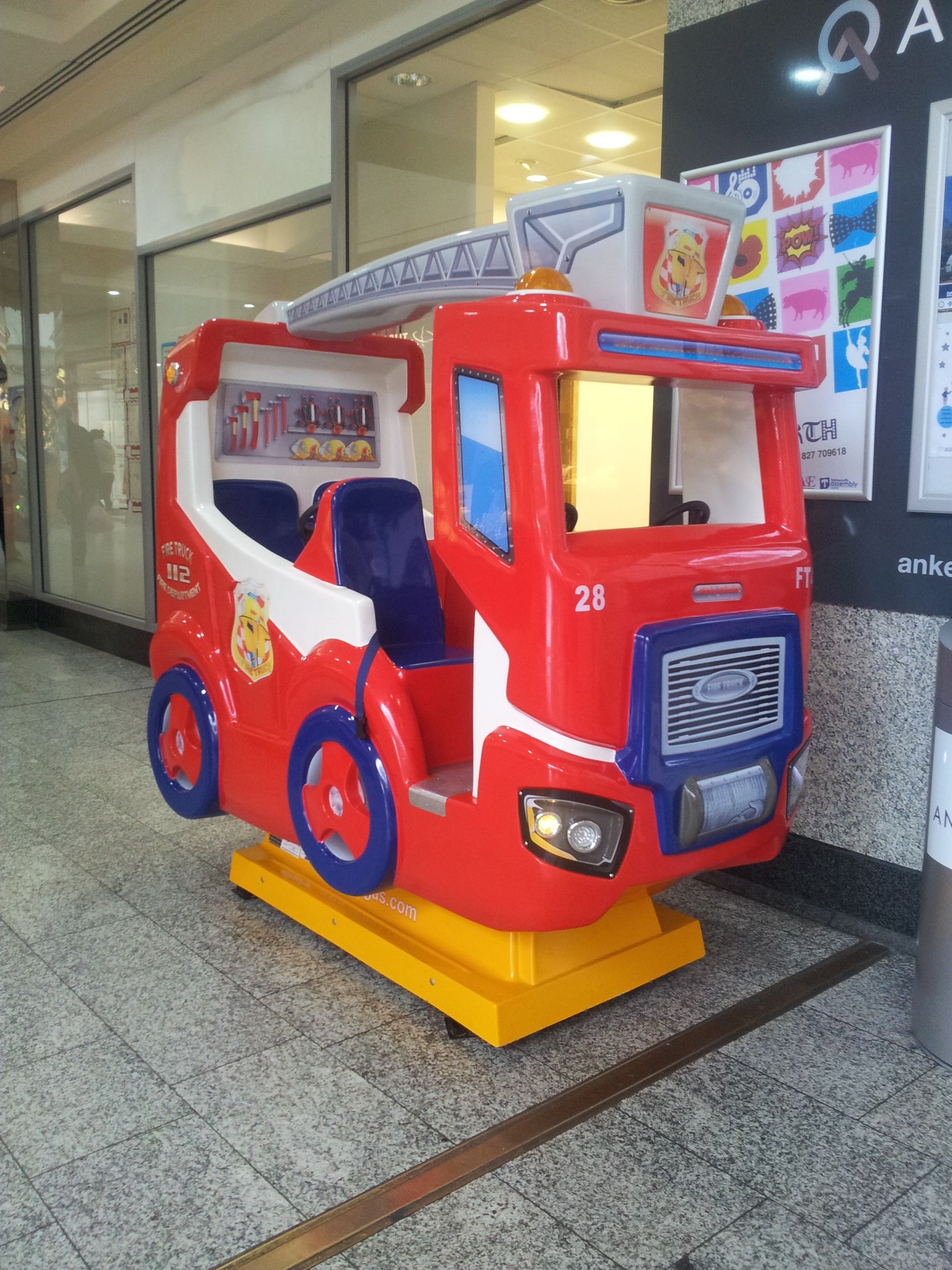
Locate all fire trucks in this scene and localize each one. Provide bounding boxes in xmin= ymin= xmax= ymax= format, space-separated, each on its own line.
xmin=147 ymin=176 xmax=827 ymax=1048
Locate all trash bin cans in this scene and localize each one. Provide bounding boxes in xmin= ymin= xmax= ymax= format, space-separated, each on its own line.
xmin=908 ymin=615 xmax=951 ymax=1075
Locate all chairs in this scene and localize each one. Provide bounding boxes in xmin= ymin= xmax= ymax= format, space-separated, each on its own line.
xmin=212 ymin=479 xmax=301 ymax=571
xmin=317 ymin=479 xmax=472 ymax=758
